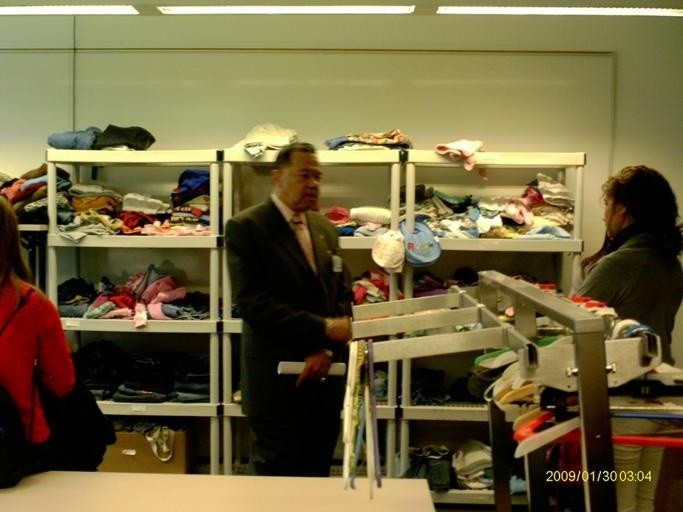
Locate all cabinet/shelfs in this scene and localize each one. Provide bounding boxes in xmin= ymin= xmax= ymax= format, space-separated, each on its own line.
xmin=43 ymin=148 xmax=587 ymax=506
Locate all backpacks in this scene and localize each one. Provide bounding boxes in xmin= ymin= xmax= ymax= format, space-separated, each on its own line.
xmin=0 ymin=287 xmax=41 ymax=488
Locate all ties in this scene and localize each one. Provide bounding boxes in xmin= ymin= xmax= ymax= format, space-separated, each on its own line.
xmin=291 ymin=215 xmax=316 ymax=275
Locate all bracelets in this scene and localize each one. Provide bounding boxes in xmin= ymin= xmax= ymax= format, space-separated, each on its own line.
xmin=322 ymin=349 xmax=334 ymax=358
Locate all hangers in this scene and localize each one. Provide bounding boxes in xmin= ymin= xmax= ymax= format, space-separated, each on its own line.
xmin=473 ymin=291 xmax=682 ymax=458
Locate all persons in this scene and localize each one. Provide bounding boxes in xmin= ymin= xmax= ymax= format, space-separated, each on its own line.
xmin=0 ymin=194 xmax=76 ymax=476
xmin=224 ymin=143 xmax=355 ymax=477
xmin=573 ymin=164 xmax=683 ymax=512
